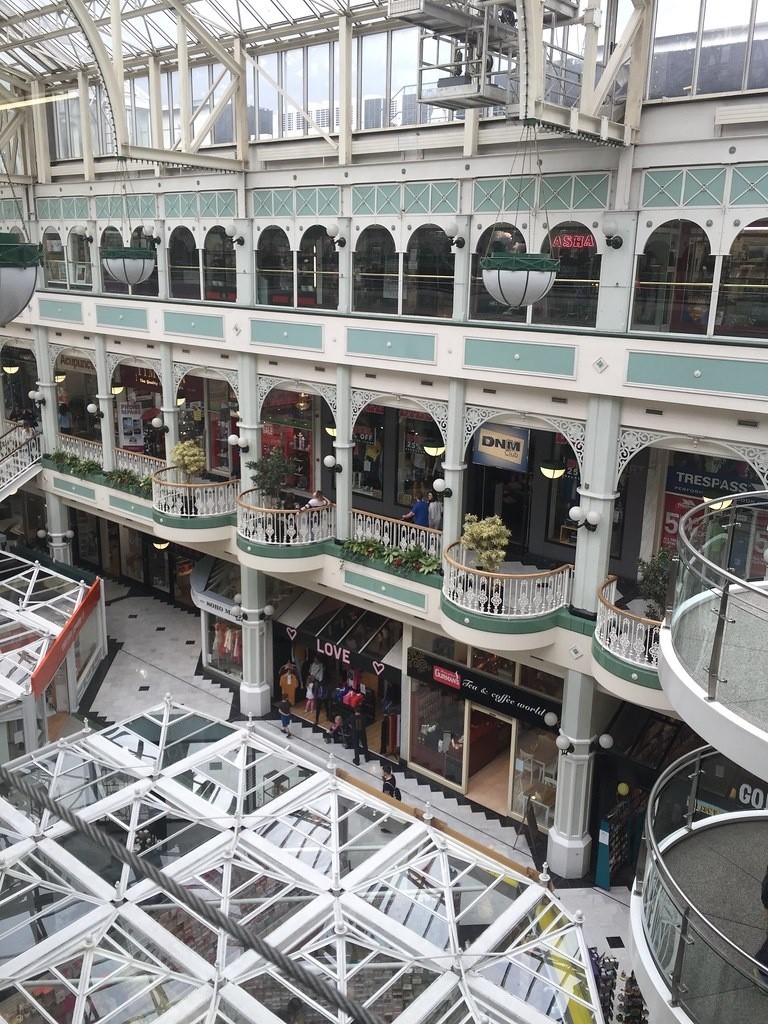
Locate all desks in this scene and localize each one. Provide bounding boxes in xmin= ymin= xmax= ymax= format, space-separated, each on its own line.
xmin=521 ymin=782 xmax=556 ymax=828
xmin=529 ymin=744 xmax=557 ymax=785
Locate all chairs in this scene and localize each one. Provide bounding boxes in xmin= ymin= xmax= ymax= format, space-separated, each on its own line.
xmin=518 ymin=734 xmax=559 ymax=791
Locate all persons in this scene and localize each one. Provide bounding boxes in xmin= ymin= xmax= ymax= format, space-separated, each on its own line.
xmin=278 ymin=693 xmax=294 ymax=739
xmin=323 ymin=716 xmax=343 ymax=735
xmin=344 ymin=703 xmax=369 ymax=766
xmin=304 ymin=656 xmax=329 ymax=727
xmin=403 ymin=490 xmax=442 ymax=529
xmin=284 ymin=492 xmax=300 ymax=519
xmin=379 ymin=765 xmax=397 ymax=834
xmin=295 ymin=490 xmax=331 ymax=526
xmin=57 ymin=403 xmax=72 ymax=434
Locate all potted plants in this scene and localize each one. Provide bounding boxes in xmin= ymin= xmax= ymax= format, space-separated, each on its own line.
xmin=172 ymin=438 xmax=206 ymax=517
xmin=637 ymin=545 xmax=673 ymax=666
xmin=459 ymin=513 xmax=512 ymax=616
xmin=244 ymin=446 xmax=298 ymax=545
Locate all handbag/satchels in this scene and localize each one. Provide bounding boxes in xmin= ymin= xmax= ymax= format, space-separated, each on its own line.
xmin=312 ymin=682 xmax=329 ymax=701
xmin=384 ymin=781 xmax=401 ymax=801
xmin=341 ymin=728 xmax=352 ymax=749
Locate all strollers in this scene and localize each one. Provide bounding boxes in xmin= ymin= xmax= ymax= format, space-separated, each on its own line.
xmin=322 ymin=713 xmax=353 ymax=750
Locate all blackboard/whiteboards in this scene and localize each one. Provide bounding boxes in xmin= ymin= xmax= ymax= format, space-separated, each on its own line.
xmin=444 ymin=754 xmax=462 ymax=787
xmin=515 ymin=800 xmax=540 ymax=845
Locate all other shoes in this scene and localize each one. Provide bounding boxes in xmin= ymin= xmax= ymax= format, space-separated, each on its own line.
xmin=365 ymin=756 xmax=368 ymax=761
xmin=281 ymin=729 xmax=286 ymax=733
xmin=353 ymin=758 xmax=359 ymax=765
xmin=381 ymin=828 xmax=391 ymax=833
xmin=287 ymin=732 xmax=291 ymax=738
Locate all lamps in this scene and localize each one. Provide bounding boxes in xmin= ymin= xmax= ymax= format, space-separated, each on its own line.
xmin=151 ymin=417 xmax=170 ymax=436
xmin=325 ymin=415 xmax=338 ymax=437
xmin=432 ymin=479 xmax=453 ymax=499
xmin=54 ymin=358 xmax=66 ymax=383
xmin=422 ymin=421 xmax=445 ymax=457
xmin=0 ymin=122 xmax=768 ymax=333
xmin=111 ymin=370 xmax=124 ymax=395
xmin=227 ymin=434 xmax=250 ymax=453
xmin=87 ymin=404 xmax=104 ymax=420
xmin=230 ymin=594 xmax=274 ymax=622
xmin=702 ymin=452 xmax=734 ymax=510
xmin=323 ymin=455 xmax=342 ymax=474
xmin=28 ymin=390 xmax=46 ymax=407
xmin=538 ymin=434 xmax=567 ymax=480
xmin=36 ymin=529 xmax=74 ymax=544
xmin=544 ymin=711 xmax=613 ymax=757
xmin=615 ymin=782 xmax=630 ymax=801
xmin=2 ymin=361 xmax=18 ymax=375
xmin=152 ymin=538 xmax=170 ymax=550
